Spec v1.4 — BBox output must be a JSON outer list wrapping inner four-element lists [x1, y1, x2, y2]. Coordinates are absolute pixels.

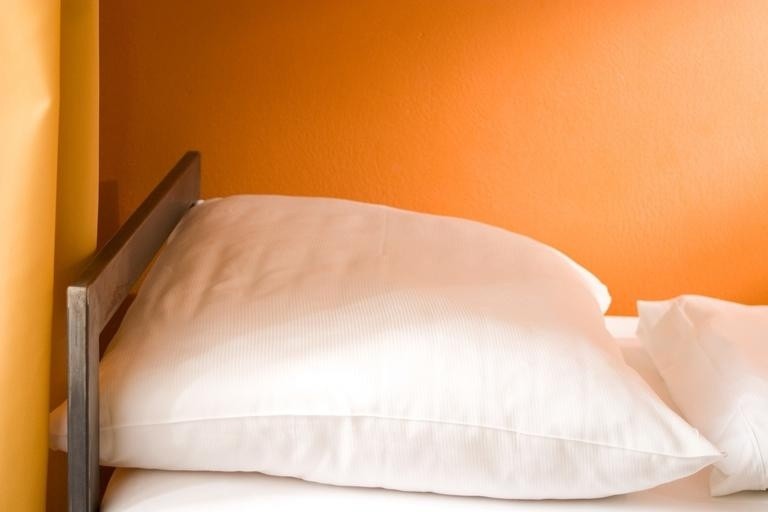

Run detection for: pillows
[[637, 295, 768, 496], [50, 194, 725, 497]]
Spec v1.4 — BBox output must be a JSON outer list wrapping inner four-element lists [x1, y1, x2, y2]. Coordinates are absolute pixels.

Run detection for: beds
[[67, 151, 767, 510]]
